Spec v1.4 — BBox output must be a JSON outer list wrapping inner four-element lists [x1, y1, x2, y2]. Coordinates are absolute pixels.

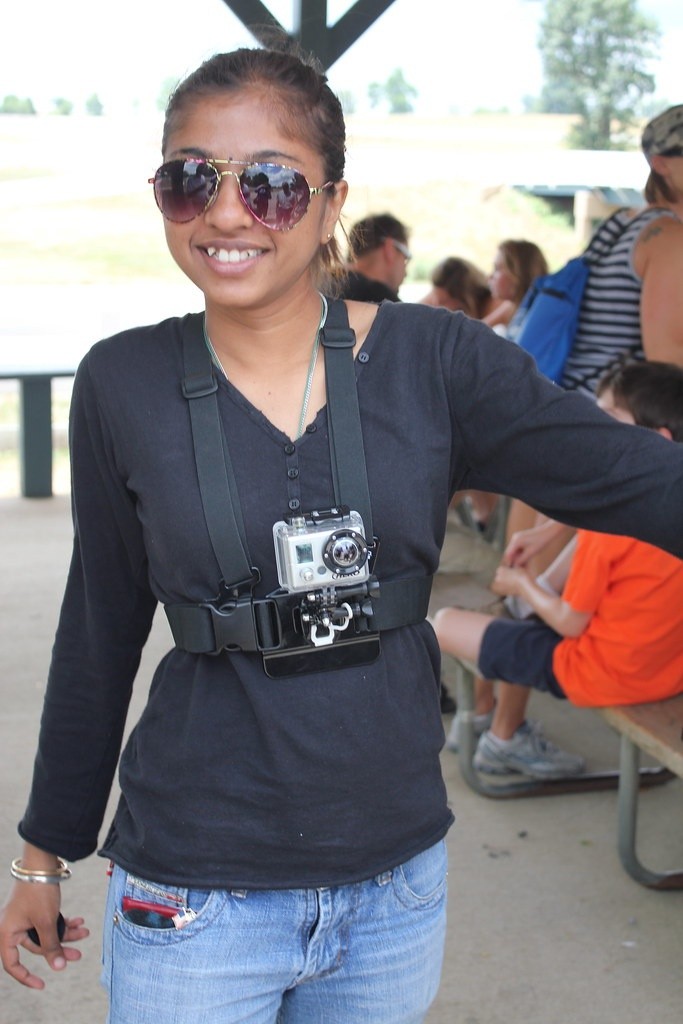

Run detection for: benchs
[[482, 602, 683, 891]]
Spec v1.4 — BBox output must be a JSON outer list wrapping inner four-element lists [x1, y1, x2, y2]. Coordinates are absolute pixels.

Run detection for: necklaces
[[202, 293, 328, 438]]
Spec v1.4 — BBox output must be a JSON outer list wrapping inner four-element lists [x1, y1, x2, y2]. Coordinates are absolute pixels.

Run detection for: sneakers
[[471, 724, 585, 778], [446, 711, 546, 752]]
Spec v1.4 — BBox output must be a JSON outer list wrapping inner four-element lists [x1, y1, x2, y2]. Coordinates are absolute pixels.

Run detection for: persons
[[420, 104, 683, 778], [329, 213, 408, 303], [0, 48, 683, 1024]]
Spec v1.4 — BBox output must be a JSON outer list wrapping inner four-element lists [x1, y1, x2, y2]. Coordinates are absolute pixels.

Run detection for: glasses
[[148, 159, 335, 232], [382, 237, 412, 266]]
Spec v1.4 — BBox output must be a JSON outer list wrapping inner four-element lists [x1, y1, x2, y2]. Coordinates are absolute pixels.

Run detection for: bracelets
[[10, 857, 71, 883]]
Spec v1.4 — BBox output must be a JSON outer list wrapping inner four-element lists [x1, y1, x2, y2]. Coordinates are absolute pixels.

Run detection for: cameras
[[272, 505, 370, 595]]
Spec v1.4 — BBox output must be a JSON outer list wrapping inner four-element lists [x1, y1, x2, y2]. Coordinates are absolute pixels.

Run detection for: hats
[[642, 105, 683, 159]]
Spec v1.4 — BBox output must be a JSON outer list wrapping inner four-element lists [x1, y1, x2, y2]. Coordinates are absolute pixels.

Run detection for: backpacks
[[504, 213, 644, 385]]
